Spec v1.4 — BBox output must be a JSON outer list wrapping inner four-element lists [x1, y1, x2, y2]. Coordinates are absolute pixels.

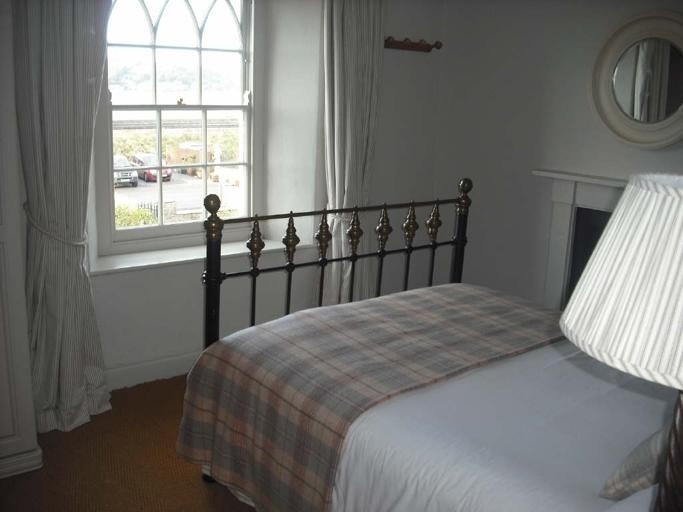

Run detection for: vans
[[130, 151, 172, 182]]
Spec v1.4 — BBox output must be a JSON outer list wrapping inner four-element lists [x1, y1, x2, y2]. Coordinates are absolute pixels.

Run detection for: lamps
[[559, 175, 683, 512]]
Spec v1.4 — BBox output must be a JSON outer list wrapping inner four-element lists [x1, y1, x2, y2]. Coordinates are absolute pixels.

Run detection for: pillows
[[597, 415, 672, 502]]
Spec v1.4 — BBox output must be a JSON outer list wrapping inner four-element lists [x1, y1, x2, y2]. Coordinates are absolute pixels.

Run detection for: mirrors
[[591, 12, 683, 149]]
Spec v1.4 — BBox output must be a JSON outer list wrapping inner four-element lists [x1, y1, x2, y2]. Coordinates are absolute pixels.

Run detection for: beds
[[199, 179, 682, 512]]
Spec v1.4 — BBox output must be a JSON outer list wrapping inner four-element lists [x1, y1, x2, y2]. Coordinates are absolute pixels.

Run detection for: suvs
[[114, 154, 137, 187]]
